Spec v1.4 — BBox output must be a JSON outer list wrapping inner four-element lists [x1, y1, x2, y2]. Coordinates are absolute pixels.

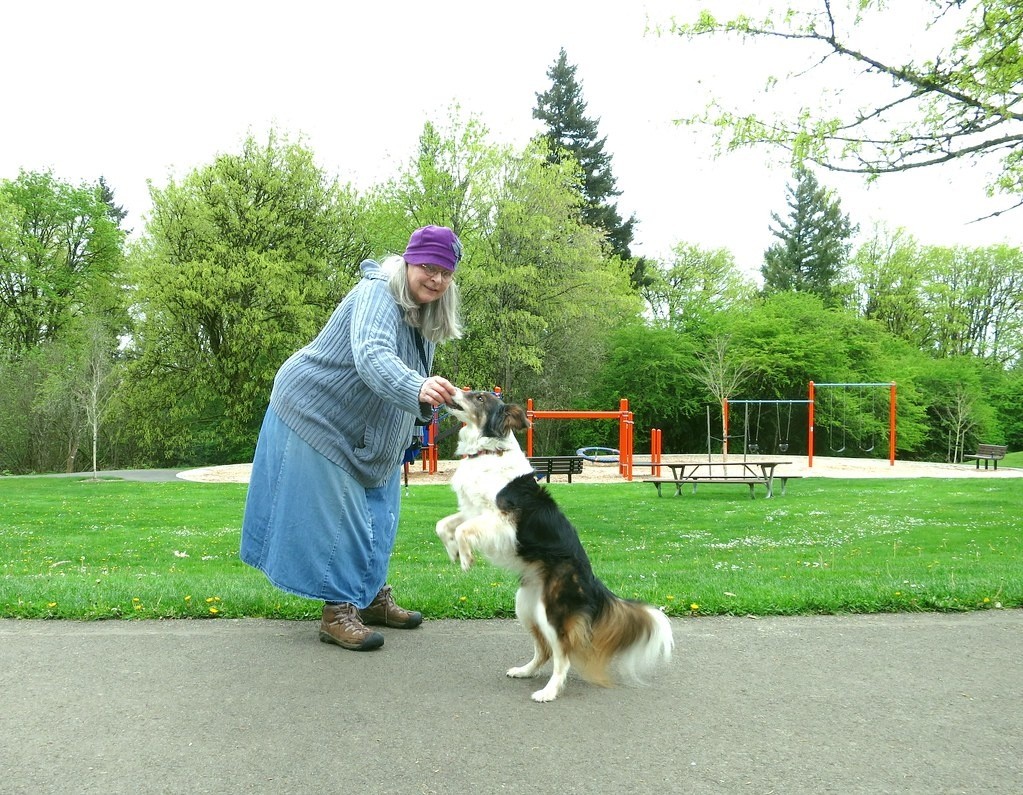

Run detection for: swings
[[830, 385, 846, 453], [747, 402, 762, 454], [859, 385, 875, 453], [776, 401, 792, 453]]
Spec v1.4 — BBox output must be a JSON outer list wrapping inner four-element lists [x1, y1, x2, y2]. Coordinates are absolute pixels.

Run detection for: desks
[[649, 461, 792, 499]]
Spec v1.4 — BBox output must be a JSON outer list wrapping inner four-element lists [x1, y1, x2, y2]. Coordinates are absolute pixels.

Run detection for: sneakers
[[319, 603, 384, 651], [357, 586, 422, 629]]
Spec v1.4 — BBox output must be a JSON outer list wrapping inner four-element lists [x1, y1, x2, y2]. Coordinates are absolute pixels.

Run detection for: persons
[[239, 224, 463, 649]]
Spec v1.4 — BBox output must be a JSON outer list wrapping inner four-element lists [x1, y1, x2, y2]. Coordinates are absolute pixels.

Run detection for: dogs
[[435, 386, 675, 702]]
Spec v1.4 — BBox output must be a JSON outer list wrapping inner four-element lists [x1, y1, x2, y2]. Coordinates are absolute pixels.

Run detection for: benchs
[[686, 475, 803, 497], [642, 478, 768, 500], [524, 456, 584, 484], [963, 443, 1007, 470]]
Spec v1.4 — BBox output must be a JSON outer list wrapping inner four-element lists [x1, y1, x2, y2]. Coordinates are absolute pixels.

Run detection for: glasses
[[422, 264, 453, 283]]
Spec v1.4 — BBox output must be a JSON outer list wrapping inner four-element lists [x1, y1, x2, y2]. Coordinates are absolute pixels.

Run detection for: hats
[[402, 225, 463, 272]]
